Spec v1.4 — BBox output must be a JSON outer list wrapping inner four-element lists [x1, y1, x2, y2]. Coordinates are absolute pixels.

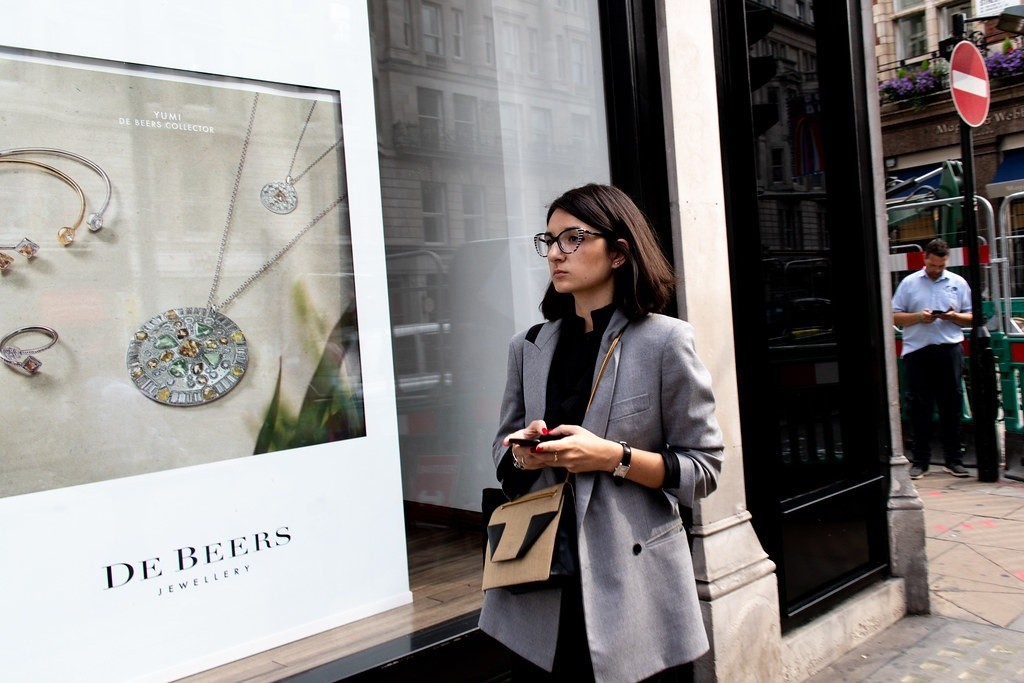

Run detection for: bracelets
[[917, 312, 922, 322], [514, 453, 523, 470], [0, 147, 112, 269]]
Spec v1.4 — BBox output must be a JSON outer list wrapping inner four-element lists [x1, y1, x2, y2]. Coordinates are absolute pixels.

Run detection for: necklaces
[[261, 100, 343, 214], [127, 93, 345, 406]]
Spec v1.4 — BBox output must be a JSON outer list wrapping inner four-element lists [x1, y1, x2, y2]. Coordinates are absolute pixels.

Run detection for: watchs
[[613, 441, 632, 481]]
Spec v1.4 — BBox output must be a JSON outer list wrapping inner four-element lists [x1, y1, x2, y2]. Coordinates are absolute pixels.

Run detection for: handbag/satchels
[[482, 483, 563, 589]]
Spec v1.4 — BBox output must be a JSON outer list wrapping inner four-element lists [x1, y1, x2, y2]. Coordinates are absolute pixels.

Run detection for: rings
[[554, 452, 559, 462], [522, 456, 526, 465], [0, 325, 59, 373]]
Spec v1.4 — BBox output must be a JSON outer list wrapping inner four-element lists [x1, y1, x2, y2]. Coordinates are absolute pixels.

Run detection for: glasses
[[535, 228, 608, 257]]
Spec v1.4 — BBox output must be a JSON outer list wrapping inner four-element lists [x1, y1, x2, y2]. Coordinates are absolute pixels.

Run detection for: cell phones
[[509, 438, 542, 448], [932, 310, 943, 316]]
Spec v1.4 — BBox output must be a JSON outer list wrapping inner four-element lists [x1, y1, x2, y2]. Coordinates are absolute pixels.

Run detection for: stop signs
[[948, 39, 991, 128]]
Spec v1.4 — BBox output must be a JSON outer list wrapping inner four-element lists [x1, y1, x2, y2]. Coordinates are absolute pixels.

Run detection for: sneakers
[[909, 466, 930, 478], [943, 461, 970, 475]]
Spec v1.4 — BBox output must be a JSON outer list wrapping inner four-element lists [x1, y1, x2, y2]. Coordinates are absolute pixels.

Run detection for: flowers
[[878, 60, 947, 111], [983, 37, 1024, 76]]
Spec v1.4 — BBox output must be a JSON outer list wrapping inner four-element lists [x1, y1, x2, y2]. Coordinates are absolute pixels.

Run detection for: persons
[[891, 240, 973, 477], [477, 184, 725, 683]]
